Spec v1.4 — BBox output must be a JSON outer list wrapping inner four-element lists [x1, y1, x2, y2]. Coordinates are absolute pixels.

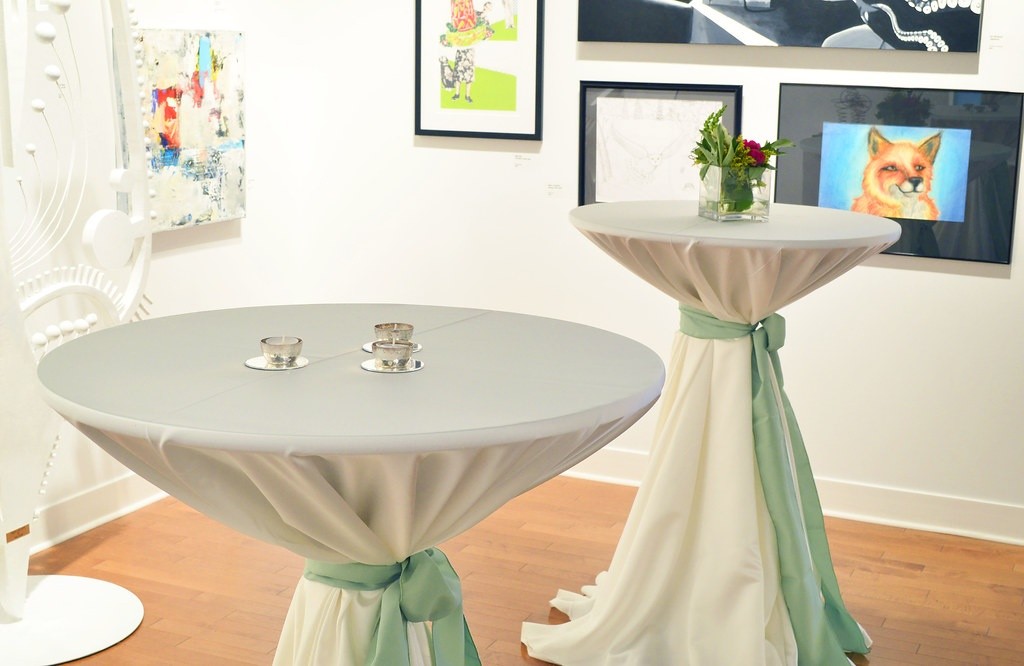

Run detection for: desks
[[35, 302, 661, 666], [523, 205, 907, 666]]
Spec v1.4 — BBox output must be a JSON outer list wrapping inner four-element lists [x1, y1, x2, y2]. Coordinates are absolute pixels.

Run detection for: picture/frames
[[414, 0, 544, 142], [576, 81, 744, 207]]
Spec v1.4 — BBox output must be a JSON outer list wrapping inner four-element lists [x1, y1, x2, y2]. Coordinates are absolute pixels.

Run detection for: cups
[[374, 322, 415, 341], [260, 336, 303, 370], [372, 340, 414, 372]]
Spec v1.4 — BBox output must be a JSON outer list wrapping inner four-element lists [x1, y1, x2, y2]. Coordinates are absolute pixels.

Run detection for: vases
[[697, 163, 772, 221]]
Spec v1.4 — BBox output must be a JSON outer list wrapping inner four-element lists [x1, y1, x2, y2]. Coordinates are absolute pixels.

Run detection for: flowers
[[691, 106, 796, 214]]
